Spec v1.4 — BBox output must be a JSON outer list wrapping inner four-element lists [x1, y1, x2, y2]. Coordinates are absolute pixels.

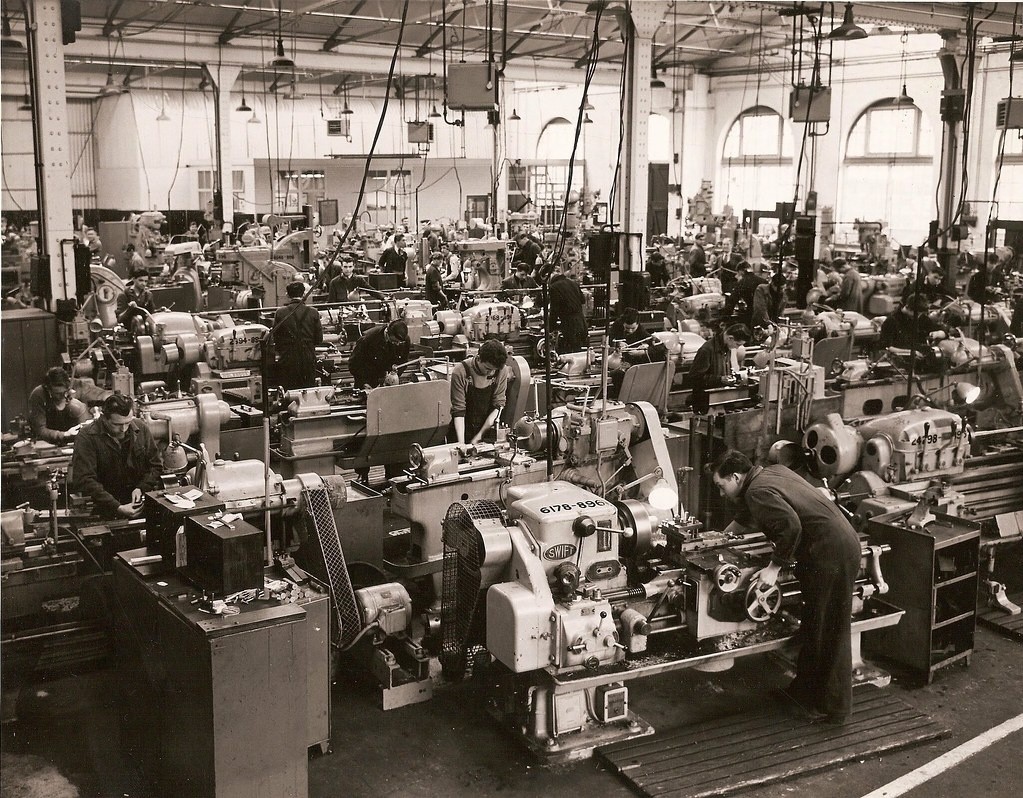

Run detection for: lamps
[[1009, 47, 1023, 61], [428, 80, 441, 117], [236, 72, 252, 111], [156, 79, 170, 121], [651, 69, 666, 88], [17, 84, 32, 111], [341, 85, 353, 113], [508, 88, 521, 120], [828, 1, 868, 40], [266, 0, 297, 69], [579, 94, 594, 110], [894, 61, 914, 104], [100, 36, 121, 94], [583, 113, 592, 122], [1, 0, 23, 48]]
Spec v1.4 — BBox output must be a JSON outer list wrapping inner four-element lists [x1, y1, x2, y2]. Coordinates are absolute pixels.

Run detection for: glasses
[[387, 332, 406, 344]]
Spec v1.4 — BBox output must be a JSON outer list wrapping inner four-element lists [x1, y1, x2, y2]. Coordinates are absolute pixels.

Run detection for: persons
[[117, 268, 156, 331], [273, 282, 323, 449], [539, 264, 590, 354], [348, 319, 410, 391], [645, 224, 1022, 375], [447, 339, 508, 446], [710, 449, 861, 724], [27, 366, 89, 447], [327, 230, 545, 321], [687, 323, 751, 530], [605, 307, 668, 398], [72, 394, 163, 616]]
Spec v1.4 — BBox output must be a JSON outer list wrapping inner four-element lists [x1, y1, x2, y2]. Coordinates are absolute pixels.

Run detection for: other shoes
[[773, 685, 810, 700], [793, 706, 845, 725]]
